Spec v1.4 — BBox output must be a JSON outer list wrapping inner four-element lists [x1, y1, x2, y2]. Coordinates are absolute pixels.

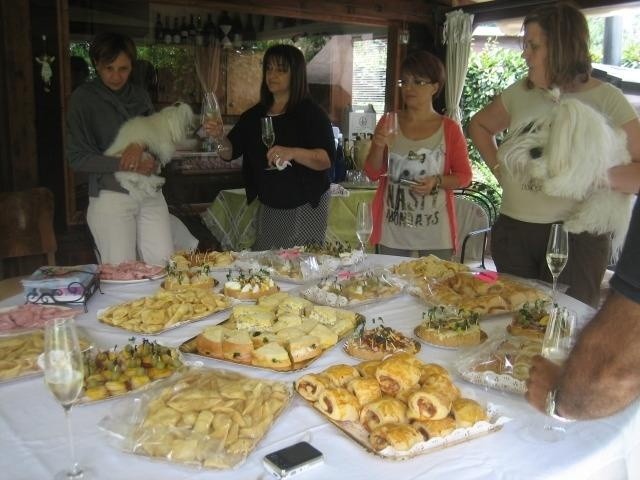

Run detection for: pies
[[295, 352, 487, 452]]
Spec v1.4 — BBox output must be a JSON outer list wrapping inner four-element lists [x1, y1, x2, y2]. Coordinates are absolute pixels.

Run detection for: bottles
[[334, 136, 362, 183]]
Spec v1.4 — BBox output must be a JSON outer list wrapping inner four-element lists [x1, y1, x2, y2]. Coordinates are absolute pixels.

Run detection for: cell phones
[[263, 440, 324, 474]]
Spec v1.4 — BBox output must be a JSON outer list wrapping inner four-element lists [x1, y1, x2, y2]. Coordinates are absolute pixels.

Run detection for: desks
[[218, 183, 383, 253]]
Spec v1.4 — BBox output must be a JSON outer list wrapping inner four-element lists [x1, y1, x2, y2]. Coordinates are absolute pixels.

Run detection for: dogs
[[103, 101, 193, 202], [496, 99, 635, 265]]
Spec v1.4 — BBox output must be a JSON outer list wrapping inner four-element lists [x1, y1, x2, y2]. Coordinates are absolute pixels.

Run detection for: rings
[[376, 124, 384, 129], [275, 154, 280, 158], [394, 131, 398, 136], [128, 164, 135, 168], [387, 127, 393, 133]]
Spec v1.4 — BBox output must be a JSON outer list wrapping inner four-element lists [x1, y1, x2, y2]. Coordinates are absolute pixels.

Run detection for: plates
[[456, 332, 566, 402], [1, 335, 94, 380], [161, 279, 218, 289], [342, 337, 423, 359], [417, 290, 548, 318], [178, 309, 365, 371], [96, 291, 241, 334], [98, 263, 165, 283], [2, 305, 70, 335], [250, 254, 339, 285], [302, 269, 407, 308], [505, 316, 566, 338], [385, 255, 462, 296], [220, 286, 281, 301], [295, 360, 515, 461], [414, 322, 489, 350]]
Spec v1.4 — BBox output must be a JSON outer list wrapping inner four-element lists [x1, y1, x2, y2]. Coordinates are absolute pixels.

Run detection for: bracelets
[[492, 163, 501, 175], [428, 173, 442, 196]]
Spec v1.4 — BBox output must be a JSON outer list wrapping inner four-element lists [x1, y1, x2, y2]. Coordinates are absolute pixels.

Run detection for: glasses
[[395, 77, 434, 89]]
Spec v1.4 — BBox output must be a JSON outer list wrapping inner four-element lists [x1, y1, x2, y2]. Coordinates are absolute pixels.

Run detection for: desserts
[[164, 268, 544, 358]]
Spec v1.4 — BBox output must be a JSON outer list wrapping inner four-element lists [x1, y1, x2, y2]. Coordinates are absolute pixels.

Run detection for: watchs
[[543, 387, 578, 423]]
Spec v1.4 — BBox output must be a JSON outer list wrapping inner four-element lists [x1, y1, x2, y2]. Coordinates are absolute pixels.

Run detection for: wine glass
[[345, 169, 369, 186], [204, 90, 228, 154], [526, 308, 579, 444], [381, 112, 399, 178], [261, 116, 280, 171], [40, 316, 98, 480], [545, 222, 569, 304], [355, 198, 374, 258]]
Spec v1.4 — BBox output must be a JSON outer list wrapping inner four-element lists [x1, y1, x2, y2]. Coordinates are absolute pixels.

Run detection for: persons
[[130, 58, 160, 113], [63, 29, 175, 264], [467, 3, 640, 309], [362, 49, 473, 261], [524, 194, 640, 422], [69, 56, 90, 94], [203, 43, 336, 252]]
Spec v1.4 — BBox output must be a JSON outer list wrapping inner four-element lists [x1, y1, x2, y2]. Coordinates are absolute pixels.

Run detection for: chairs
[[1, 184, 60, 280], [451, 181, 502, 270]]
